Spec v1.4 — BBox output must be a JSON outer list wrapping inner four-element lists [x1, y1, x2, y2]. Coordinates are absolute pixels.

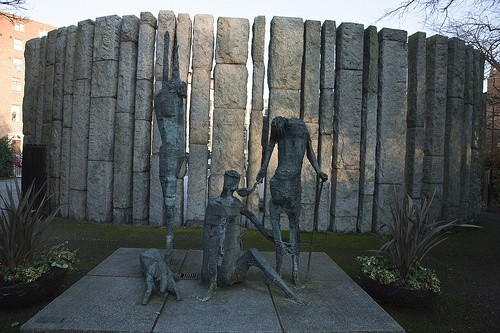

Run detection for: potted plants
[[353, 178, 485, 309], [0, 176, 60, 307], [32, 243, 79, 286]]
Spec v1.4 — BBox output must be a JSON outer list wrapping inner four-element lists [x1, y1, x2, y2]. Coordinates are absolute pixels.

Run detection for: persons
[[256, 117, 326, 288], [197, 170, 307, 305]]
